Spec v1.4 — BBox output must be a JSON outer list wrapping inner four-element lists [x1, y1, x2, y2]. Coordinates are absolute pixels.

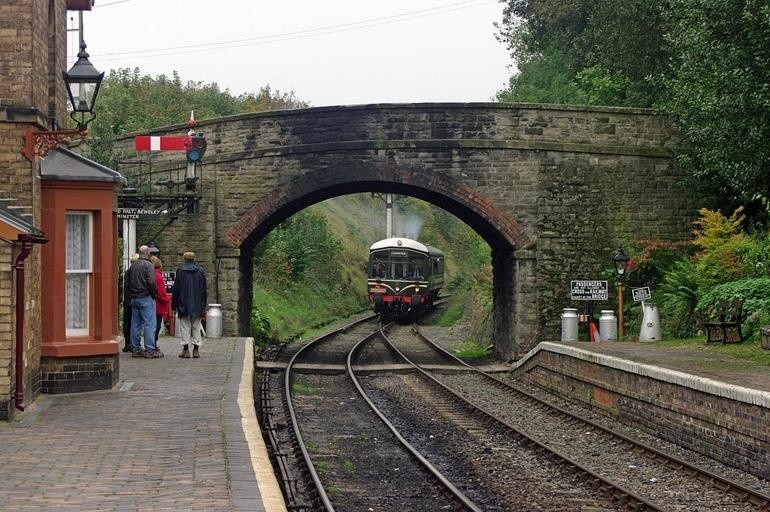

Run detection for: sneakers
[[192, 349, 200, 357], [123, 344, 164, 358], [178, 350, 189, 358]]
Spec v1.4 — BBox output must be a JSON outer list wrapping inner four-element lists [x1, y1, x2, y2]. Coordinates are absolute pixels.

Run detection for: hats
[[184, 252, 194, 259]]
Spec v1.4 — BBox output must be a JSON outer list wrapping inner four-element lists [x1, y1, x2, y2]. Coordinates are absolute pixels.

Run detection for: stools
[[760, 324, 770, 350]]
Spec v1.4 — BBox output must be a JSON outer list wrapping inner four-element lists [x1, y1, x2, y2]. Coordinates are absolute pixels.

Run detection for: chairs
[[703, 300, 745, 346]]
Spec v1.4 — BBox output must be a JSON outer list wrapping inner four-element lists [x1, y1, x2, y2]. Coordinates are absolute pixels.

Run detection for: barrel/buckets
[[175, 312, 183, 337], [205, 304, 223, 337], [561, 308, 579, 343], [599, 310, 618, 341]]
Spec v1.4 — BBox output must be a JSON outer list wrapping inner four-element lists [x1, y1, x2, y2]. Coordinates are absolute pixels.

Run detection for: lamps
[[62, 43, 105, 132]]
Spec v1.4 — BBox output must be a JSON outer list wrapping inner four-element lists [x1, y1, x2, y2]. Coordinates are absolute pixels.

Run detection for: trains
[[368, 238, 444, 319]]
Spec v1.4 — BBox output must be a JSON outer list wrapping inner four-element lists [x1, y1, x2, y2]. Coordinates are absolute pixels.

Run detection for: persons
[[121, 253, 141, 353], [171, 252, 208, 359], [411, 262, 424, 279], [434, 259, 440, 267], [148, 254, 170, 350], [128, 244, 165, 359]]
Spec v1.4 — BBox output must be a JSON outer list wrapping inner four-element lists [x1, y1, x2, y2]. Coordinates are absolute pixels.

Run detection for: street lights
[[613, 246, 630, 343]]
